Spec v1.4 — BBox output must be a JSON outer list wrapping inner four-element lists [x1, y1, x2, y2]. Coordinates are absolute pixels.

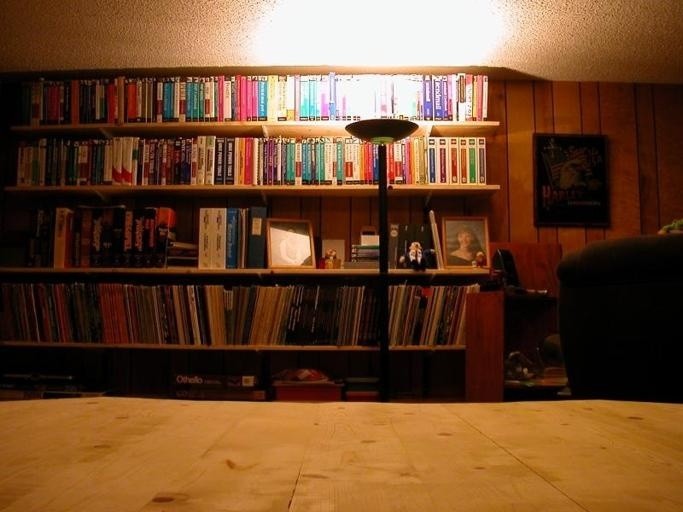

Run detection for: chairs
[[556, 233, 682, 403]]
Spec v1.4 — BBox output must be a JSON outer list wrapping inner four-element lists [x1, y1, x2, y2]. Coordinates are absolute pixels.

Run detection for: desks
[[0, 398, 683, 512]]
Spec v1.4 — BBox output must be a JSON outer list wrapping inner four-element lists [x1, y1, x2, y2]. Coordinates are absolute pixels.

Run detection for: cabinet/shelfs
[[0, 64, 506, 401]]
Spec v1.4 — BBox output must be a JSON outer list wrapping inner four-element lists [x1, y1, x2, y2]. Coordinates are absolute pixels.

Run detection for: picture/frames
[[264, 218, 317, 269], [529, 132, 615, 230], [441, 217, 489, 267]]
